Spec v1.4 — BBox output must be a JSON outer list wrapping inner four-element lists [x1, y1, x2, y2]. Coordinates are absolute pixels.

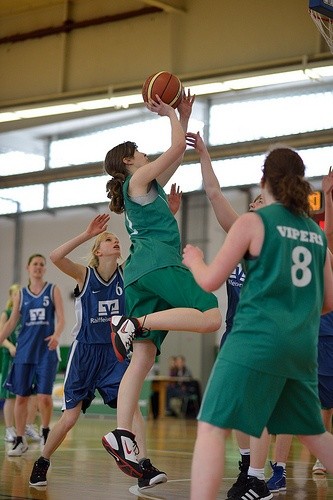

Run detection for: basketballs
[[141, 70, 183, 115]]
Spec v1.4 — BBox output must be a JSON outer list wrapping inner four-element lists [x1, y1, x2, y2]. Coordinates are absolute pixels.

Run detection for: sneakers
[[110, 314, 151, 362], [226, 454, 250, 497], [227, 476, 274, 500], [5, 429, 15, 441], [29, 457, 51, 485], [8, 436, 29, 457], [266, 461, 286, 492], [137, 459, 169, 490], [312, 460, 327, 474], [101, 428, 142, 477], [25, 424, 41, 440], [39, 426, 51, 451]]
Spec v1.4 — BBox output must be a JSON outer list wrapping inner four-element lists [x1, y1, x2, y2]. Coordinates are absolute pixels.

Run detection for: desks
[[149, 376, 194, 419]]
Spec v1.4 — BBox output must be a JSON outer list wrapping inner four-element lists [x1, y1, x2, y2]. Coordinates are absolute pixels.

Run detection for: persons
[[103, 87, 221, 479], [0, 255, 63, 457], [150, 357, 192, 417], [181, 133, 333, 500], [29, 183, 182, 491]]
